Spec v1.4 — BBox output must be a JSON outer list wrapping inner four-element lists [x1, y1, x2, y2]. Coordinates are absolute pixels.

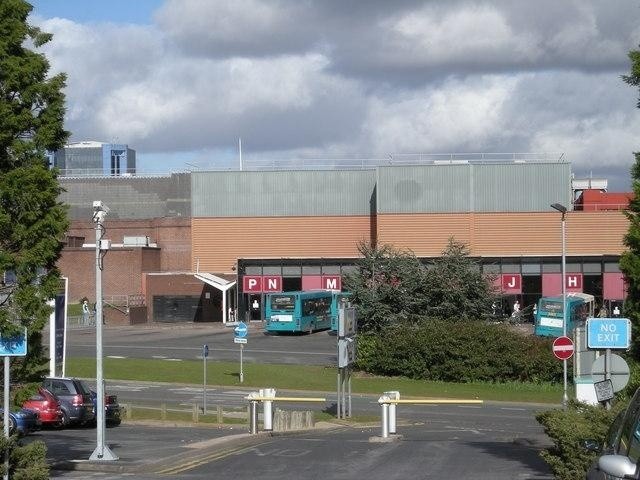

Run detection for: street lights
[[551, 203, 570, 411]]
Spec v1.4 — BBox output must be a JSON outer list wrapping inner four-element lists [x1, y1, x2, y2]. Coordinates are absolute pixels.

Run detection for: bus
[[329, 290, 354, 335], [264, 287, 341, 335], [534, 291, 596, 340]]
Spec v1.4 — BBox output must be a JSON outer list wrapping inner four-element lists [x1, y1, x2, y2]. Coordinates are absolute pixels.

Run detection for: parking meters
[[258, 388, 278, 431], [383, 390, 401, 434]]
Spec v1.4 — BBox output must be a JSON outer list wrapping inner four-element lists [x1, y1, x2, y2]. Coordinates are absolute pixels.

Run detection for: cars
[[88, 388, 122, 428], [580, 408, 627, 480], [508, 302, 535, 326], [1, 403, 42, 439]]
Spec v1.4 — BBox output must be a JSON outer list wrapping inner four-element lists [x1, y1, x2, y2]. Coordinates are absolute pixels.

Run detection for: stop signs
[[551, 335, 575, 360]]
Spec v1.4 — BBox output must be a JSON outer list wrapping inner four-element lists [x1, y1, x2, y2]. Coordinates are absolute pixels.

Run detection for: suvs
[[597, 385, 640, 480], [0, 382, 65, 432], [34, 377, 96, 429]]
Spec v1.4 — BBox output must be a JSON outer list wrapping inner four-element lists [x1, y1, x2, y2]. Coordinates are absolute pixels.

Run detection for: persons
[[83, 300, 105, 324], [513, 300, 522, 327], [532, 303, 538, 326], [571, 304, 620, 320]]
